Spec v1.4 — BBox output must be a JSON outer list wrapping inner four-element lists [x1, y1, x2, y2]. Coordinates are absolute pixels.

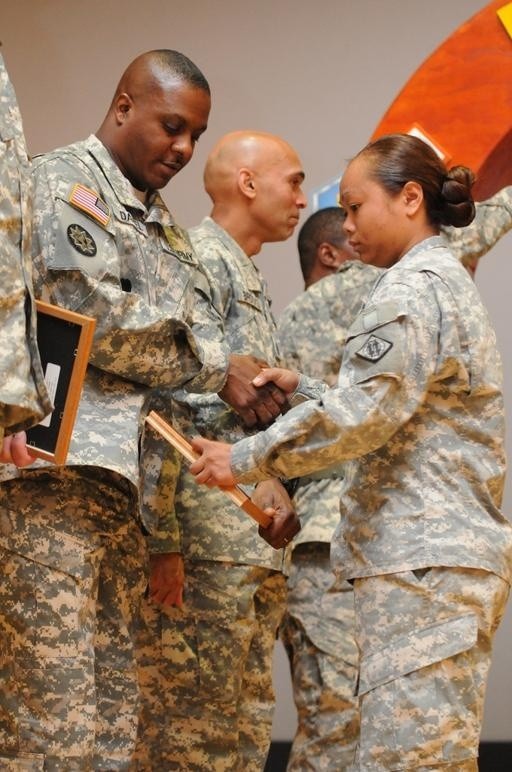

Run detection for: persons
[[1, 41, 304, 771], [182, 127, 510, 771], [0, 53, 56, 496], [271, 166, 510, 770], [146, 124, 310, 771]]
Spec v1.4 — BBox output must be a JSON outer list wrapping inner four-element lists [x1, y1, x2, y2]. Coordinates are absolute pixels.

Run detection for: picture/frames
[[13, 299, 97, 466], [144, 406, 277, 532]]
